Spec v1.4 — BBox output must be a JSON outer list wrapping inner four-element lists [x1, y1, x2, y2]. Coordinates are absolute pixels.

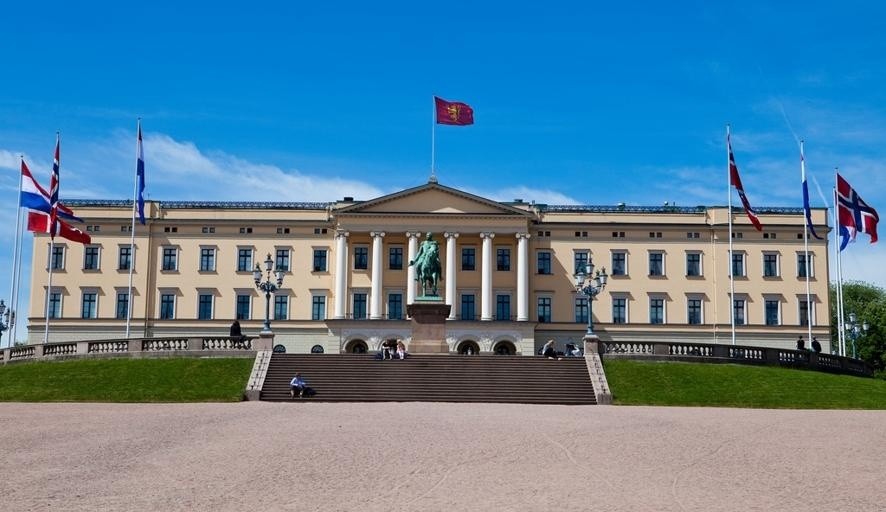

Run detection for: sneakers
[[548, 357, 563, 361], [383, 357, 404, 360]]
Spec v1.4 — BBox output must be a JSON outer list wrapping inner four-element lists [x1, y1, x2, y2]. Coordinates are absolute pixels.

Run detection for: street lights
[[0, 298, 15, 346], [251, 252, 285, 332], [844, 313, 869, 359], [575, 258, 608, 335]]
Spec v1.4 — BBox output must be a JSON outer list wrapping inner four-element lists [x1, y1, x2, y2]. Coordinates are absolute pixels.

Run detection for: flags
[[18, 158, 83, 222], [835, 171, 880, 244], [833, 188, 856, 251], [799, 145, 824, 241], [433, 93, 475, 126], [46, 138, 59, 242], [26, 207, 90, 246], [726, 134, 761, 231], [136, 124, 145, 225]]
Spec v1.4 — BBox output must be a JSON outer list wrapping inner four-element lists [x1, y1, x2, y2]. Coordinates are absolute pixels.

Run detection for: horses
[[420, 241, 440, 298]]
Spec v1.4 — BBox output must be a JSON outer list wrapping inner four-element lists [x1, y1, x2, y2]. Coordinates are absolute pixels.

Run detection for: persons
[[406, 231, 443, 282], [542, 340, 563, 361], [380, 338, 393, 361], [809, 335, 821, 353], [793, 334, 804, 352], [287, 372, 306, 400], [562, 337, 576, 357], [395, 338, 406, 361], [229, 320, 244, 343]]
